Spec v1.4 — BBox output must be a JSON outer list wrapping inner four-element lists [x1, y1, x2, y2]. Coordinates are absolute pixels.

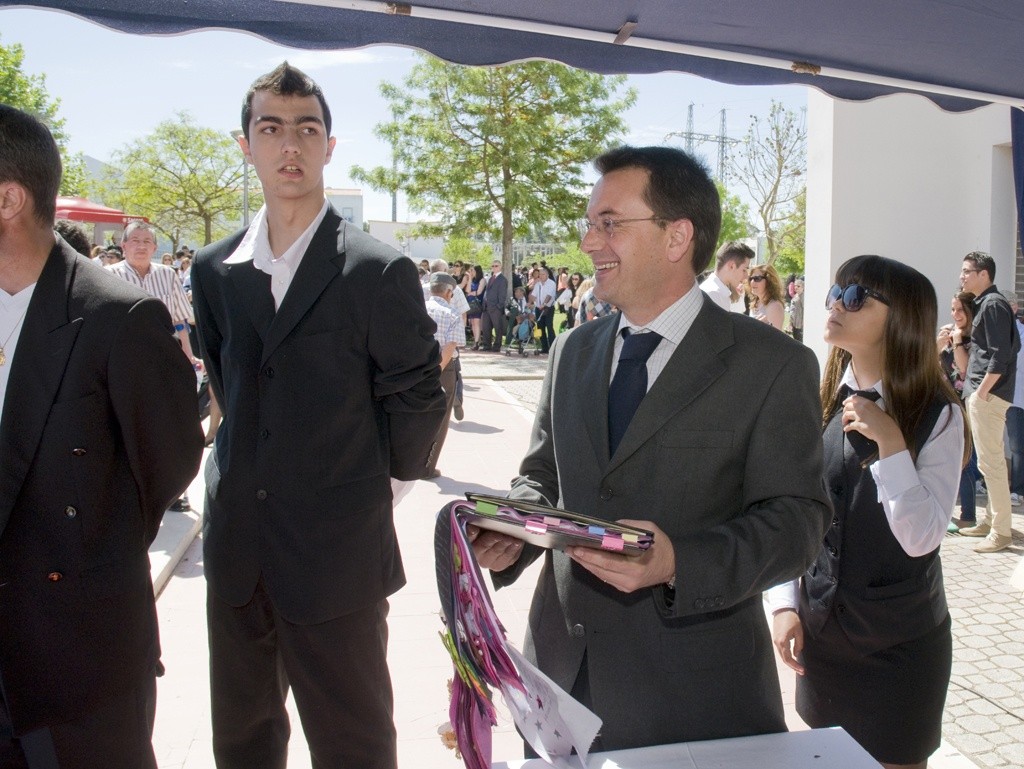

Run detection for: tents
[[54, 196, 149, 225]]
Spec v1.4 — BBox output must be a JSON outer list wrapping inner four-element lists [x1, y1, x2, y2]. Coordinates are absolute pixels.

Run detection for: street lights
[[229, 129, 249, 228]]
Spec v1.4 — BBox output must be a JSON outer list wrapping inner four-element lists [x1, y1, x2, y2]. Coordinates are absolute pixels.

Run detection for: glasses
[[826, 283, 890, 311], [571, 278, 578, 280], [453, 265, 459, 267], [748, 276, 766, 281], [492, 265, 499, 267], [961, 269, 977, 274], [448, 288, 454, 297], [577, 217, 665, 238]]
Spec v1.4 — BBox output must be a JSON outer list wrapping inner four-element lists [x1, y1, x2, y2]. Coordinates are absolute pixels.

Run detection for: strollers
[[505, 305, 547, 358]]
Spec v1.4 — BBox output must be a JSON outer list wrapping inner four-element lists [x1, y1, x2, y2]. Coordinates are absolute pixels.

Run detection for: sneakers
[[975, 479, 988, 496], [959, 522, 992, 536], [1010, 493, 1023, 506], [973, 531, 1012, 552]]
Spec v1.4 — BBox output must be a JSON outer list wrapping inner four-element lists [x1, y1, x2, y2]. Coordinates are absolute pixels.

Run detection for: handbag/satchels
[[466, 290, 482, 314]]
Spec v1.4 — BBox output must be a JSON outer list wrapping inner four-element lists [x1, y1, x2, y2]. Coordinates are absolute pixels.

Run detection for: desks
[[489, 725, 886, 769]]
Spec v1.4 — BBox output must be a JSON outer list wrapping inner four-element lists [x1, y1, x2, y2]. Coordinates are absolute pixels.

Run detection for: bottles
[[194, 364, 202, 393]]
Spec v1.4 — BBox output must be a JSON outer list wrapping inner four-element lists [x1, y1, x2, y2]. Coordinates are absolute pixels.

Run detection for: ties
[[843, 389, 881, 461], [607, 325, 661, 459], [488, 276, 495, 288]]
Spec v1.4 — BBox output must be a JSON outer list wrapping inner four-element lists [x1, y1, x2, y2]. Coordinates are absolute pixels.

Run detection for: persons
[[695, 241, 805, 344], [765, 254, 964, 769], [53, 221, 222, 514], [191, 60, 448, 769], [464, 145, 833, 759], [959, 251, 1022, 552], [997, 290, 1024, 506], [937, 288, 980, 532], [412, 259, 619, 422], [0, 102, 204, 769]]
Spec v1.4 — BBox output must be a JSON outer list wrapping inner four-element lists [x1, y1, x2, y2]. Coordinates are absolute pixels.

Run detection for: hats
[[431, 271, 456, 290], [514, 287, 526, 295]]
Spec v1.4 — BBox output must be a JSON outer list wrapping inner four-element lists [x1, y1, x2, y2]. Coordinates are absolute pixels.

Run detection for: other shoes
[[171, 499, 191, 512], [540, 349, 549, 355], [946, 519, 976, 532], [480, 349, 491, 351], [453, 398, 464, 420], [490, 349, 499, 352], [471, 343, 479, 350]]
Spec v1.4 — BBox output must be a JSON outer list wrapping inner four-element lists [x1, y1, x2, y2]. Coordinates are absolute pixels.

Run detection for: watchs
[[543, 303, 546, 305], [951, 342, 963, 350]]
